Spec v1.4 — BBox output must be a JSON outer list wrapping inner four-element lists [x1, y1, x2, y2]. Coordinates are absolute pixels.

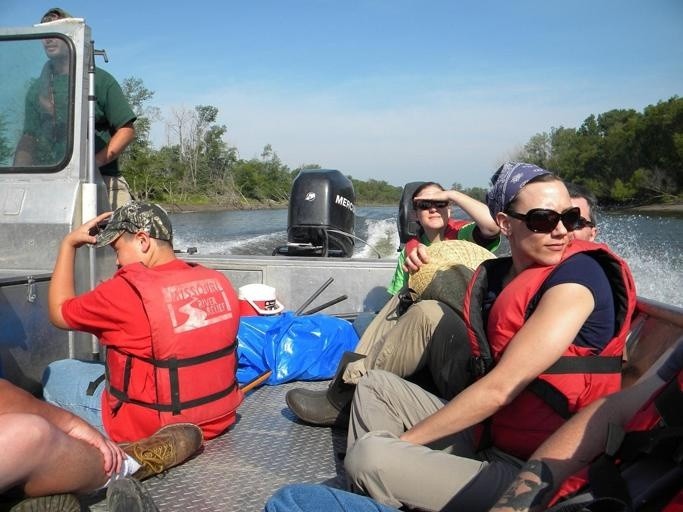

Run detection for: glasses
[[507, 207, 580, 234], [415, 199, 449, 209]]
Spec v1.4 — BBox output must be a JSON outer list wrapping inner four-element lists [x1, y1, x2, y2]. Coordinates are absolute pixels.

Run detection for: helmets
[[577, 216, 594, 229]]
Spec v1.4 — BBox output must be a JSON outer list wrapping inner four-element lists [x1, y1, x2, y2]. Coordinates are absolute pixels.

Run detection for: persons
[[43, 200, 243, 454], [12, 6, 142, 215], [335, 154, 638, 510], [0, 369, 205, 498], [350, 179, 504, 346], [283, 190, 600, 429], [262, 332, 682, 512]]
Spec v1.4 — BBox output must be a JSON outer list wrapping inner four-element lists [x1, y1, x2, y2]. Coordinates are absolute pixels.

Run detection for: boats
[[1, 18, 681, 511]]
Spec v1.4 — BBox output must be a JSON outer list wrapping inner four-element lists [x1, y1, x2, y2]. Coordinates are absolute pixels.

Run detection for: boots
[[286, 351, 366, 425]]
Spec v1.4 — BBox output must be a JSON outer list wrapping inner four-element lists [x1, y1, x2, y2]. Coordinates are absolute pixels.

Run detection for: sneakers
[[7, 494, 80, 512], [106, 476, 157, 512], [118, 423, 204, 480]]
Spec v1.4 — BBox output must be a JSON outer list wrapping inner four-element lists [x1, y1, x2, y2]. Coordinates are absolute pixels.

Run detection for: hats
[[408, 240, 497, 318], [87, 201, 171, 249]]
